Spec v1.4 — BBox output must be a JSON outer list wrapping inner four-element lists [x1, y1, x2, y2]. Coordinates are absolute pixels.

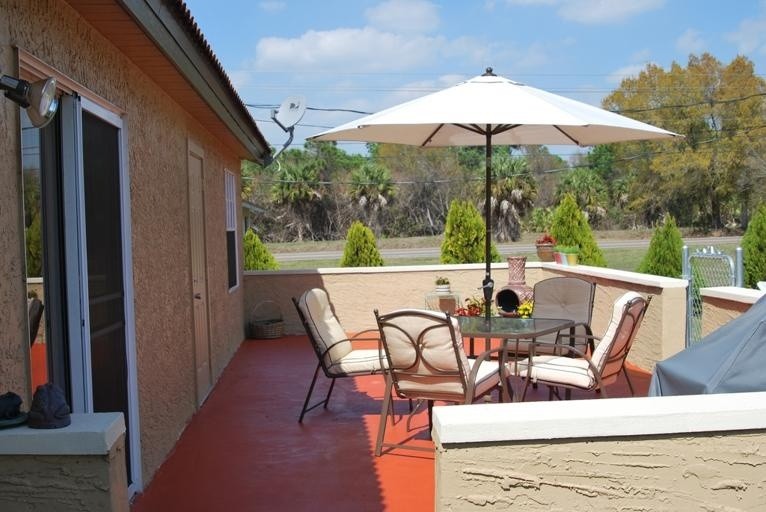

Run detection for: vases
[[536, 244, 556, 262], [499, 254, 534, 313]]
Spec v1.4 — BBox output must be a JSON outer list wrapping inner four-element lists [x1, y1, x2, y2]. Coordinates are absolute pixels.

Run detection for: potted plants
[[553, 246, 580, 266]]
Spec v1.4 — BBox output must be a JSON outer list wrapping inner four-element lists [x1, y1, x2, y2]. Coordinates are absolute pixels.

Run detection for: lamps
[[0, 75, 60, 128]]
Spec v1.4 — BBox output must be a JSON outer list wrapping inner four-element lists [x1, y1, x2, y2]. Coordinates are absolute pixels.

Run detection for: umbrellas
[[306, 67, 686, 360]]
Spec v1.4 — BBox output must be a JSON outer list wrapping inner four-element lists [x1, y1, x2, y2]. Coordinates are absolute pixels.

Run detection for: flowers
[[536, 234, 558, 245]]
